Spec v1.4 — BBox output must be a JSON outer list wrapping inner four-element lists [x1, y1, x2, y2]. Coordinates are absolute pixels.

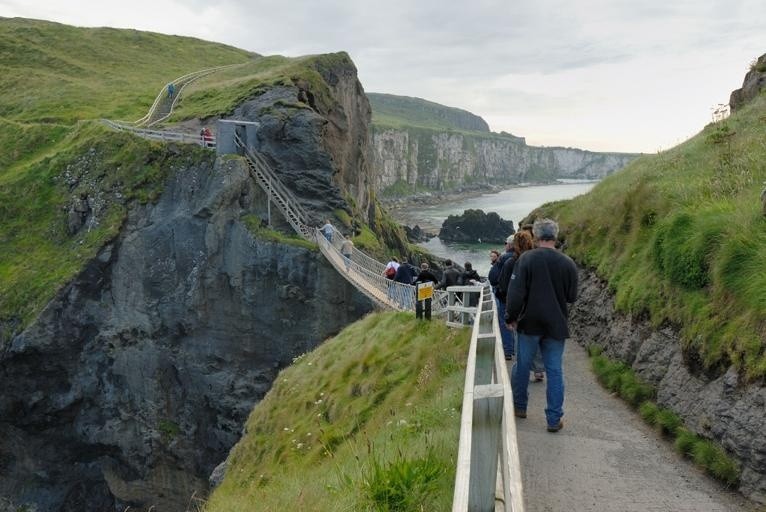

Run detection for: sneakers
[[514, 411, 527, 419], [534, 371, 544, 380], [548, 419, 563, 433]]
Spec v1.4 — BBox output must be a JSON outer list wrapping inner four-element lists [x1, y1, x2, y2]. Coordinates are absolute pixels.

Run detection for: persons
[[393, 256, 414, 311], [199, 128, 205, 140], [339, 234, 354, 273], [204, 128, 213, 147], [168, 82, 174, 99], [382, 256, 401, 302], [457, 260, 481, 324], [506, 217, 581, 433], [318, 219, 339, 251], [488, 223, 546, 381], [433, 258, 462, 307], [412, 262, 439, 287]]
